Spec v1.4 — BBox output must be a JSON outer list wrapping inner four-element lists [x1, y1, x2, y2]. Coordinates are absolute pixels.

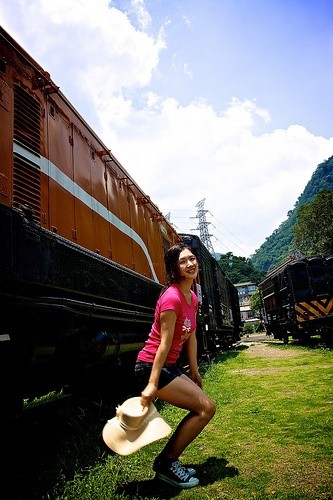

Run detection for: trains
[[0, 26, 240, 411], [255, 255, 333, 346]]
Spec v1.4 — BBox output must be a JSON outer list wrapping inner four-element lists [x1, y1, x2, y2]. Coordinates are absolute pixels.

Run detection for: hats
[[102, 397, 172, 456]]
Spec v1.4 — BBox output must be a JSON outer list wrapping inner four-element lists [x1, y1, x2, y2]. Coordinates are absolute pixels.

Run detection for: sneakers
[[153, 454, 196, 475], [157, 458, 200, 487]]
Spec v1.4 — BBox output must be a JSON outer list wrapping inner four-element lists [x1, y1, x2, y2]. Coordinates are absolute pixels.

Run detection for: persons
[[136, 242, 216, 488]]
